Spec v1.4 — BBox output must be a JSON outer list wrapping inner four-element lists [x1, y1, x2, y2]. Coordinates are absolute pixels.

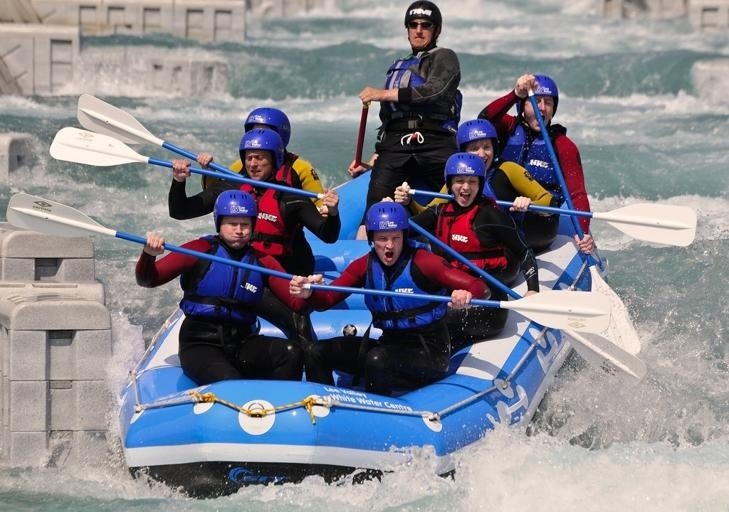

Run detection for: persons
[[169, 133, 342, 279], [347, 1, 463, 243], [135, 189, 324, 384], [288, 200, 492, 397], [395, 120, 553, 253], [394, 151, 540, 356], [476, 74, 596, 258], [195, 106, 329, 217]]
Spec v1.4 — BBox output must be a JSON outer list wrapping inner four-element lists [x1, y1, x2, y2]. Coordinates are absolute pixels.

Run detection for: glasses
[[407, 22, 431, 28]]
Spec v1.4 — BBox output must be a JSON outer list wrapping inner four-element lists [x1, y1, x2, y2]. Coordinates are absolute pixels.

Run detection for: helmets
[[516, 73, 559, 117], [245, 107, 290, 147], [404, 0, 442, 30], [365, 202, 411, 245], [456, 119, 501, 155], [214, 190, 258, 232], [239, 128, 285, 170], [445, 153, 485, 191]]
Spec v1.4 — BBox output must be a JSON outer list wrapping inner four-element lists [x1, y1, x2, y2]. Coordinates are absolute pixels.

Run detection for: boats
[[116, 168, 602, 501]]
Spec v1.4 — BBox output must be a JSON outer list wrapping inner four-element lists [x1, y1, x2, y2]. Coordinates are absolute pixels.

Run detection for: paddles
[[77, 93, 245, 176], [408, 218, 646, 389], [50, 127, 324, 200], [527, 88, 641, 354], [409, 188, 696, 245], [300, 283, 610, 332], [6, 193, 325, 284]]
